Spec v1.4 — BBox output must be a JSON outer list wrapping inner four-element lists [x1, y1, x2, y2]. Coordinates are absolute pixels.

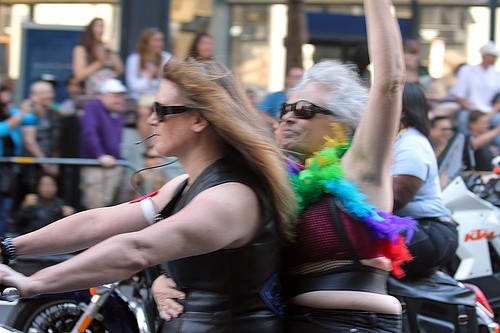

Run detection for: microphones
[[136, 133, 156, 144]]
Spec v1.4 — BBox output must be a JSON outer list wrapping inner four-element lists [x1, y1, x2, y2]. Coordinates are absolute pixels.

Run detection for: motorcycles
[[0, 254, 167, 333]]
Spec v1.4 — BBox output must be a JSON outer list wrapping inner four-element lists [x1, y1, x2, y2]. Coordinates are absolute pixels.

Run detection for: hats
[[97, 78, 127, 94], [480, 42, 499, 57]]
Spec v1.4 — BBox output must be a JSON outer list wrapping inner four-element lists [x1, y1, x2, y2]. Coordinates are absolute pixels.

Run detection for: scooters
[[384, 156, 499, 332]]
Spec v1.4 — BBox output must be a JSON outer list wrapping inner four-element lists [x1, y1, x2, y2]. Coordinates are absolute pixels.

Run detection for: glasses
[[1, 99, 13, 108], [152, 102, 204, 122], [278, 100, 337, 121]]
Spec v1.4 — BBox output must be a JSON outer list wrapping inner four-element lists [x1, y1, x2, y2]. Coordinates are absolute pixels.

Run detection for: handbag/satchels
[[387, 273, 479, 333]]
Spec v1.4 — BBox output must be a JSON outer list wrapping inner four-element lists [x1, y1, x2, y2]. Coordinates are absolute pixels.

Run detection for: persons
[[0, 72, 82, 239], [75, 79, 127, 209], [0, 58, 298, 332], [71, 18, 125, 84], [402, 40, 422, 83], [256, 62, 304, 121], [427, 42, 500, 208], [392, 83, 459, 280], [131, 144, 168, 201], [150, 0, 406, 332], [188, 32, 216, 60], [124, 25, 174, 104]]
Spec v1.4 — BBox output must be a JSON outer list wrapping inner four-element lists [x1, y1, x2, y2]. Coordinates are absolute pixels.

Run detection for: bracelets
[[3, 237, 16, 260]]
